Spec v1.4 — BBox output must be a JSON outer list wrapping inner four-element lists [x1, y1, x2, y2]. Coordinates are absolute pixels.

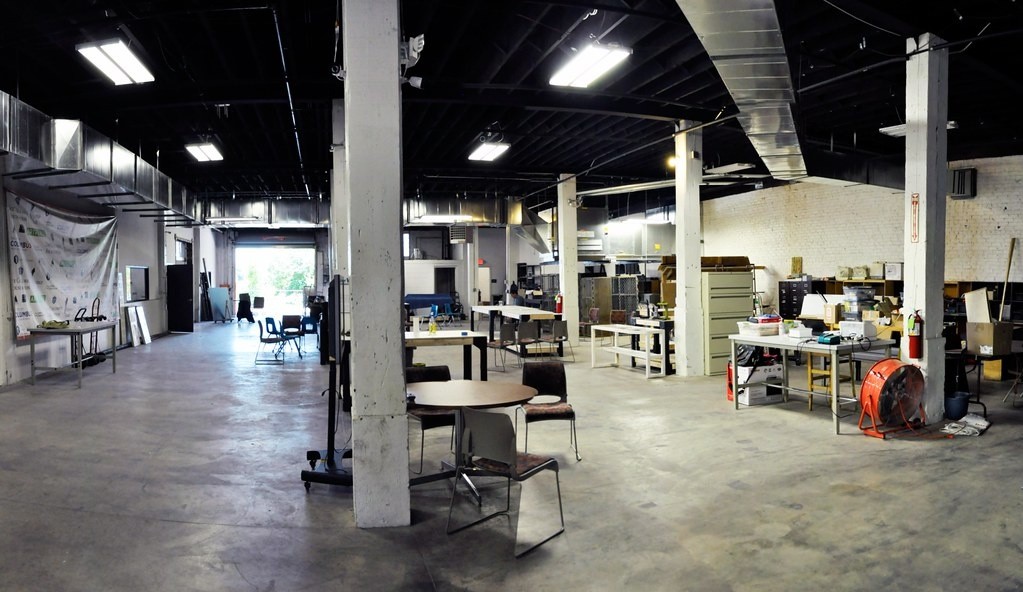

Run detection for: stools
[[808, 352, 858, 412]]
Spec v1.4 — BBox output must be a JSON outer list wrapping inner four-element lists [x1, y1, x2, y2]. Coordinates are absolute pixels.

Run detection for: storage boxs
[[885, 262, 904, 281], [966, 322, 1014, 356], [727, 363, 783, 405], [870, 263, 885, 280], [845, 299, 879, 312], [844, 286, 876, 301]]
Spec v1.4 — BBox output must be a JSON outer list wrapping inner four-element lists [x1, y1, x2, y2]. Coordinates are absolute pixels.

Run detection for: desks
[[406, 380, 538, 505], [471, 305, 564, 357], [405, 330, 488, 381], [26, 319, 118, 387], [728, 332, 896, 436]]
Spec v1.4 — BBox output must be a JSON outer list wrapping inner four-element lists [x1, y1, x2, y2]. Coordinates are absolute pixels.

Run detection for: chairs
[[514, 362, 582, 464], [255, 314, 319, 365], [580, 308, 604, 341], [441, 303, 462, 328], [419, 304, 442, 330], [404, 365, 456, 475], [610, 310, 631, 346], [503, 322, 544, 367], [538, 321, 576, 363], [445, 410, 566, 558], [488, 323, 521, 372]]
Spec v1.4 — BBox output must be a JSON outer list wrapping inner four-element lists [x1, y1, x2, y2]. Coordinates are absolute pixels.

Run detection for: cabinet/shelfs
[[779, 280, 1023, 354]]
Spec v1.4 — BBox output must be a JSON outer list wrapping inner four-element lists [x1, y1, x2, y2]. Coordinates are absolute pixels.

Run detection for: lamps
[[185, 133, 224, 161], [468, 131, 513, 161], [548, 39, 634, 88], [74, 29, 155, 86]]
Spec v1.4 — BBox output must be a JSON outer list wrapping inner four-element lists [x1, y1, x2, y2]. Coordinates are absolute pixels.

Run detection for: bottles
[[428, 312, 437, 335]]
